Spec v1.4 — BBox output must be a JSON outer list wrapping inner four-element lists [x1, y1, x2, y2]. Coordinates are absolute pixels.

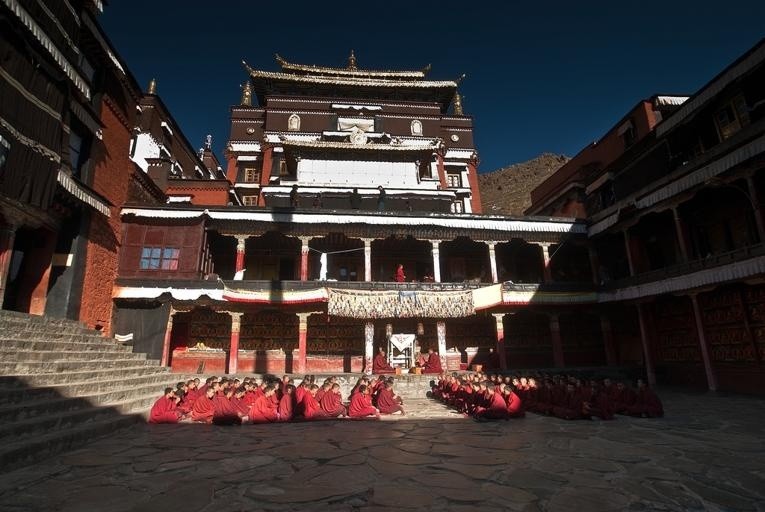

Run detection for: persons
[[290, 184, 301, 207], [424, 271, 434, 282], [422, 348, 441, 369], [351, 187, 361, 209], [395, 263, 407, 282], [149, 372, 404, 425], [431, 370, 665, 424], [377, 185, 385, 211], [373, 351, 393, 370], [312, 193, 321, 208]]
